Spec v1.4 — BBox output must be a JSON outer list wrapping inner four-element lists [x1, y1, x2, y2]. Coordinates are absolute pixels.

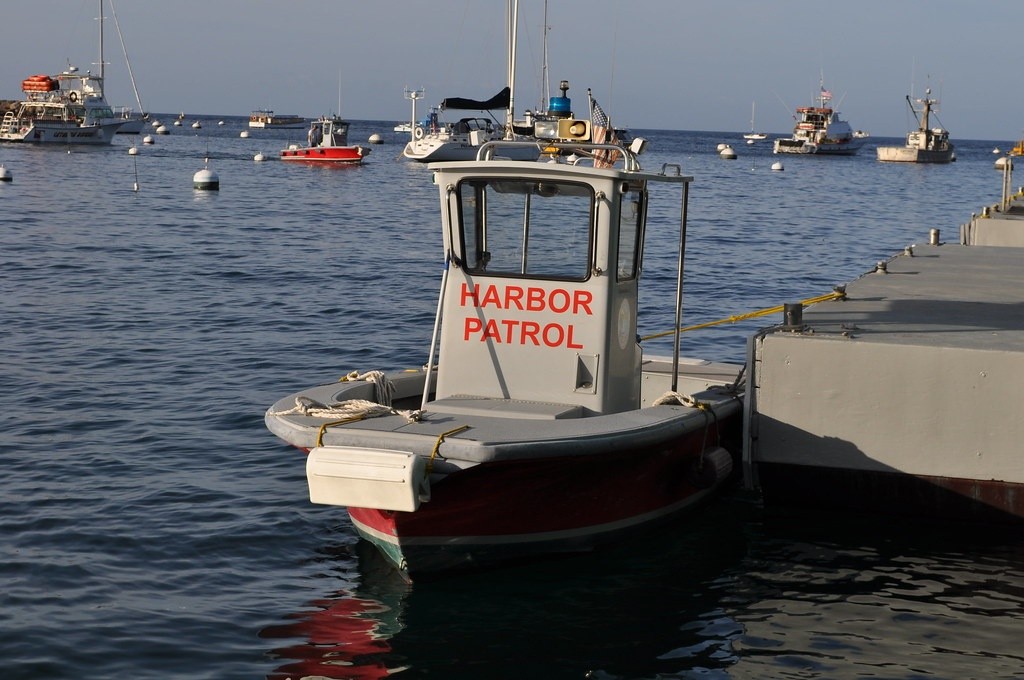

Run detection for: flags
[[820, 87, 832, 98], [589, 97, 623, 169]]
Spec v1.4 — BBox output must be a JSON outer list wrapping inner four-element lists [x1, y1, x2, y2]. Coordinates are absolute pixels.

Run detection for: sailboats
[[99, 1, 152, 134], [743, 100, 768, 140]]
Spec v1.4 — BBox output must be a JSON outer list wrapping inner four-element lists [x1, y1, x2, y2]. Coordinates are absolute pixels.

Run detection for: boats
[[403, 1, 632, 163], [0, 62, 136, 144], [875, 72, 955, 164], [394, 120, 419, 132], [248, 109, 306, 130], [262, 141, 716, 588], [773, 64, 870, 157], [277, 110, 374, 162]]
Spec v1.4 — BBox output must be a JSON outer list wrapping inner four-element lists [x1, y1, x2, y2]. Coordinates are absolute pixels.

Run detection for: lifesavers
[[415, 127, 423, 140], [28, 74, 50, 82], [252, 115, 257, 120], [69, 91, 78, 104]]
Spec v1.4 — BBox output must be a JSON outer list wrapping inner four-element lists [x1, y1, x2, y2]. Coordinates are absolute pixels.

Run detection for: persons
[[337, 125, 344, 132], [308, 124, 318, 147], [316, 125, 322, 145]]
